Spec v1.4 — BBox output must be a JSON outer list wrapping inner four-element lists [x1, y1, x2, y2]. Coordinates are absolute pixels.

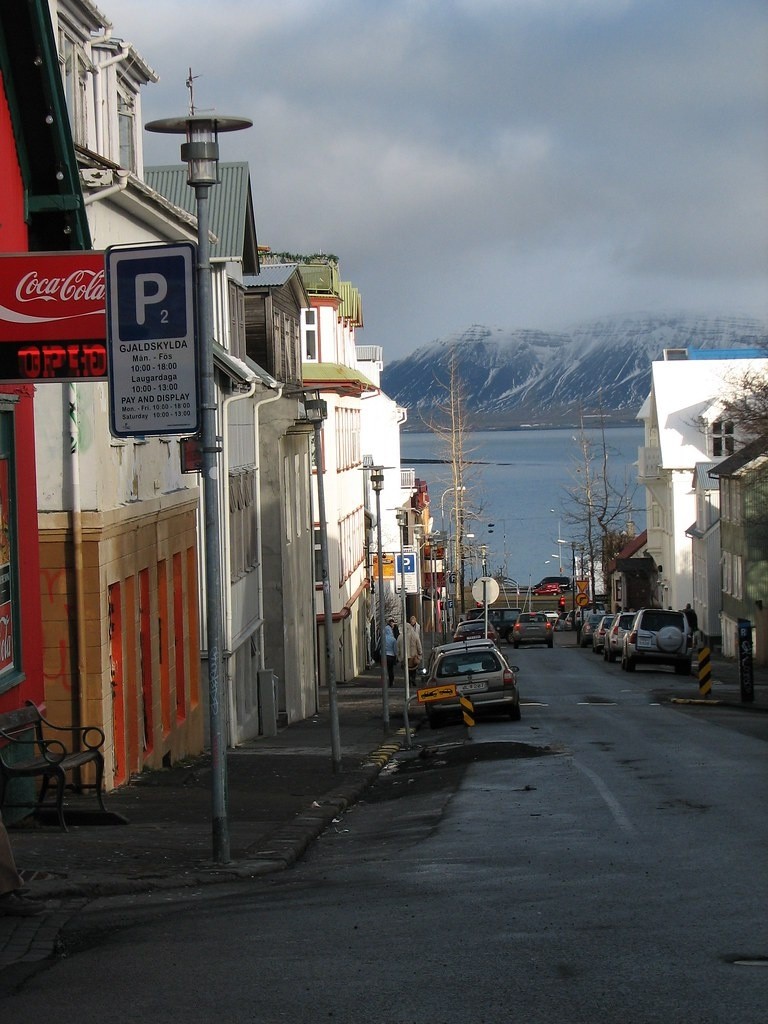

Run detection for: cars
[[428, 639, 509, 674], [552, 610, 575, 631], [423, 646, 521, 730], [580, 616, 602, 648], [531, 583, 561, 596], [604, 611, 637, 663], [593, 615, 616, 655], [453, 619, 501, 648], [512, 612, 554, 649]]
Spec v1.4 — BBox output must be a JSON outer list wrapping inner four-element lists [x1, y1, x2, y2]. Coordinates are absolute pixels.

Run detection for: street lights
[[557, 540, 592, 601], [357, 465, 396, 722], [432, 540, 443, 645], [422, 533, 440, 646], [441, 486, 466, 532], [568, 541, 577, 630], [144, 114, 255, 862]]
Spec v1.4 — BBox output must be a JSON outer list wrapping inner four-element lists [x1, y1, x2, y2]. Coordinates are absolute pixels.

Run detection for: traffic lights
[[560, 597, 565, 607]]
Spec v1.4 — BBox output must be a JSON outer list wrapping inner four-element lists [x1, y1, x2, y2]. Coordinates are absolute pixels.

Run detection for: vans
[[475, 608, 523, 645], [534, 577, 571, 590]]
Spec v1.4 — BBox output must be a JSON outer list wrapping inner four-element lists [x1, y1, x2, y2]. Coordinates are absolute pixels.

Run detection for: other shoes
[[412, 681, 416, 686]]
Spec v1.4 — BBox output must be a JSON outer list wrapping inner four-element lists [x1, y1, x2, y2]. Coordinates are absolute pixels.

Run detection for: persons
[[404, 616, 421, 636], [388, 617, 400, 641], [376, 625, 398, 688], [397, 623, 422, 686], [682, 603, 698, 631], [613, 603, 674, 612]]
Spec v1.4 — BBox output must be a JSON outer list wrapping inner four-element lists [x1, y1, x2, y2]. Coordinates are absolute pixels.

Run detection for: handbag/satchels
[[371, 650, 381, 663], [414, 654, 421, 664]]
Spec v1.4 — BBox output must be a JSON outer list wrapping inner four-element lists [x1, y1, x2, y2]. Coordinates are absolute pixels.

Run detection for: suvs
[[622, 607, 694, 676]]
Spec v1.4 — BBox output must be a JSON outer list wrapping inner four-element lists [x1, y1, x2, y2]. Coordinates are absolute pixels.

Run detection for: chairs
[[0, 700, 109, 832], [537, 617, 542, 621], [445, 662, 458, 674], [482, 659, 495, 671]]
